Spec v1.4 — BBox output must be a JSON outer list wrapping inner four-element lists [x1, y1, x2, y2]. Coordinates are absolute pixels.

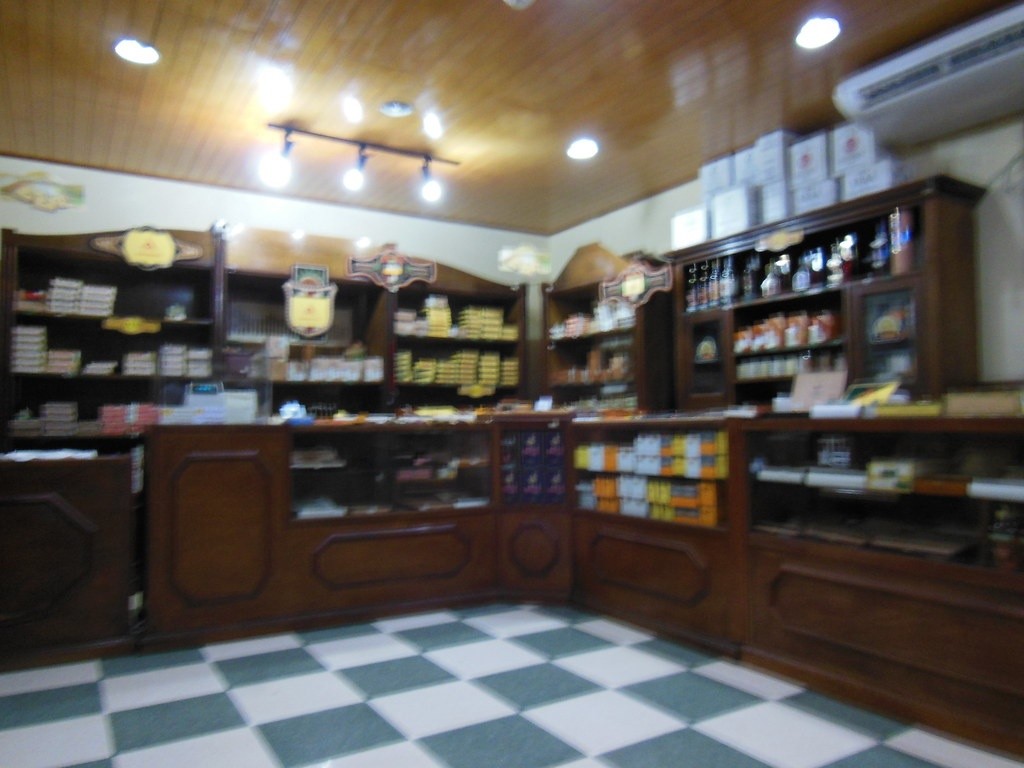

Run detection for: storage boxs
[[668, 125, 906, 251]]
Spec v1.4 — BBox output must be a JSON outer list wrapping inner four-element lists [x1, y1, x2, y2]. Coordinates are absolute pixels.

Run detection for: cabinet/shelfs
[[0, 174, 1024, 760]]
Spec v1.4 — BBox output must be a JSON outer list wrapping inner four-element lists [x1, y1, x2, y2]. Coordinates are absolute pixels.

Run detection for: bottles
[[991, 505, 1013, 560]]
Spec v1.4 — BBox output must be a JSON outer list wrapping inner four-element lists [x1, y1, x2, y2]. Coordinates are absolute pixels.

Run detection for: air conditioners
[[833, 0, 1024, 149]]
